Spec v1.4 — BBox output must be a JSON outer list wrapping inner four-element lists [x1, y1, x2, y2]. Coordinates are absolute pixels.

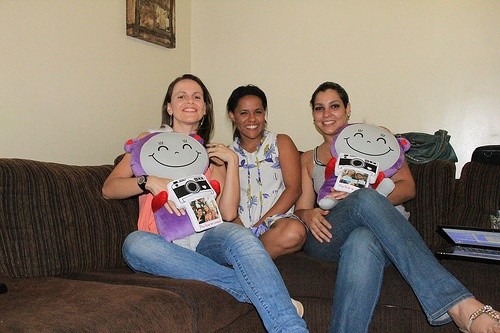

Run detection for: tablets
[[436, 225, 500, 250]]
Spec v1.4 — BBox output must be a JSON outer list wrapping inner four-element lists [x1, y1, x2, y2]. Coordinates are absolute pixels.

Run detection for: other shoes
[[290, 297, 305, 317]]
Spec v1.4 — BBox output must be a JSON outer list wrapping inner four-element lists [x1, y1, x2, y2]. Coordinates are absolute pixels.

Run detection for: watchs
[[136, 175, 150, 193]]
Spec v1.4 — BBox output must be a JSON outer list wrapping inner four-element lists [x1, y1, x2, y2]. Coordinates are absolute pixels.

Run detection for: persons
[[203, 204, 218, 221], [196, 208, 205, 223], [100, 72, 311, 333], [293, 81, 500, 333], [227, 84, 308, 261], [341, 169, 370, 189]]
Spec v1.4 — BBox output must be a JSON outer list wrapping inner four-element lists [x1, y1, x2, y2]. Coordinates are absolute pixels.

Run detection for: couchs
[[0, 144, 500, 333]]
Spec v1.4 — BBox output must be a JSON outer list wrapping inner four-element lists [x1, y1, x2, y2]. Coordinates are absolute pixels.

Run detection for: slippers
[[456, 305, 500, 333]]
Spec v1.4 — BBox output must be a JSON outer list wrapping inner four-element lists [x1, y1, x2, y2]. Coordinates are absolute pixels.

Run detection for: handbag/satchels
[[392, 129, 458, 165]]
